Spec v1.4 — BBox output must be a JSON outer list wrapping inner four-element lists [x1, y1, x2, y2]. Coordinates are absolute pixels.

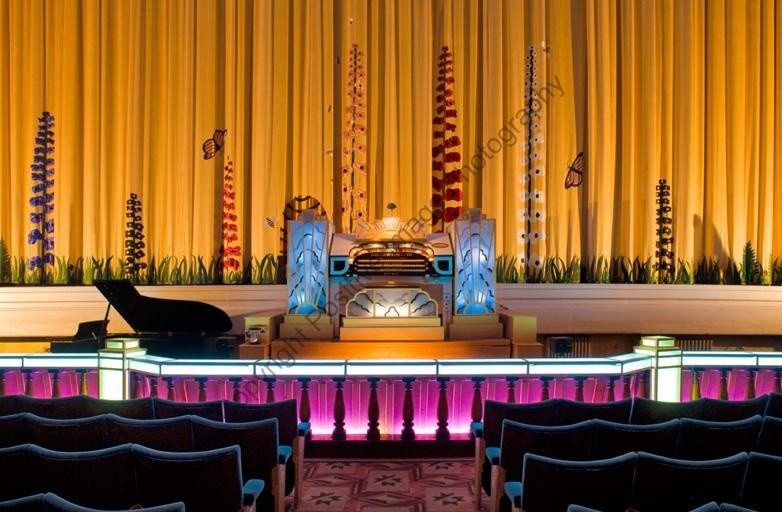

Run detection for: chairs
[[26, 413, 106, 449], [42, 492, 185, 512], [700, 394, 769, 421], [153, 398, 223, 423], [471, 398, 559, 505], [675, 415, 763, 459], [0, 493, 42, 512], [0, 395, 153, 419], [31, 442, 132, 492], [633, 451, 749, 512], [559, 397, 633, 425], [720, 504, 755, 512], [630, 398, 705, 426], [566, 500, 720, 512], [592, 418, 681, 459], [224, 399, 311, 506], [765, 391, 782, 418], [481, 418, 592, 512], [0, 413, 26, 445], [106, 413, 190, 451], [190, 415, 296, 512], [756, 416, 782, 455], [741, 451, 782, 512], [504, 452, 637, 512], [0, 443, 31, 495], [132, 444, 265, 512]]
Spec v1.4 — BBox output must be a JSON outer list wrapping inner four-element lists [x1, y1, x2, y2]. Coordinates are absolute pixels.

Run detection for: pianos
[[91, 277, 234, 357]]
